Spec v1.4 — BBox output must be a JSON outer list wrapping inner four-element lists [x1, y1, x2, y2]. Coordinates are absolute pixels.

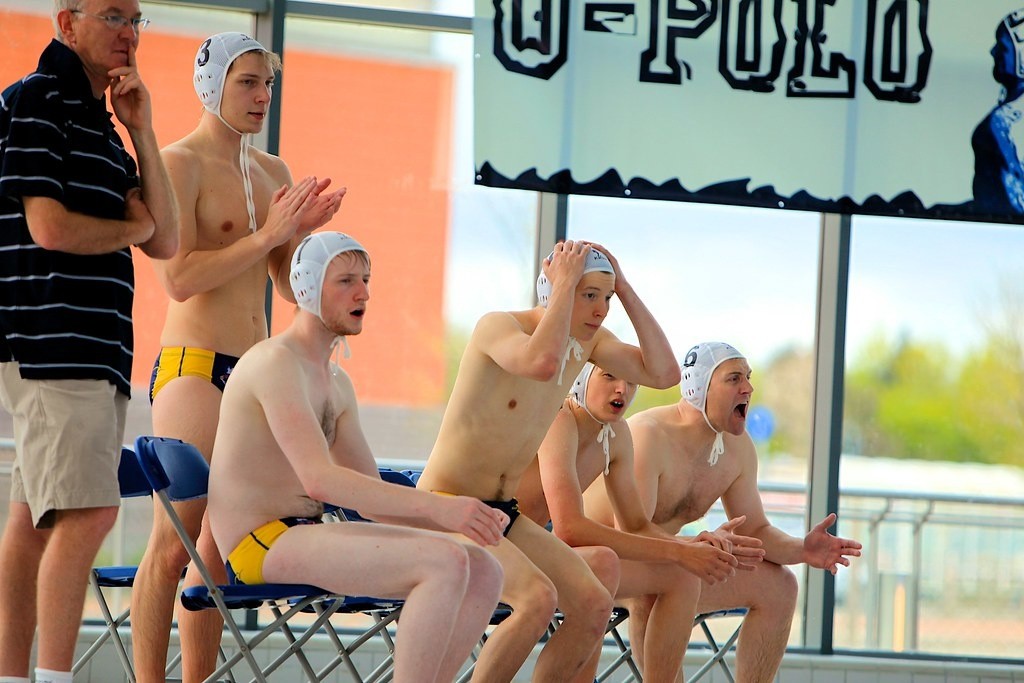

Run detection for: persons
[[415, 238, 684, 683], [514, 361, 737, 682], [580, 340, 861, 683], [207, 232, 512, 682], [0, 0, 177, 683], [127, 30, 344, 682]]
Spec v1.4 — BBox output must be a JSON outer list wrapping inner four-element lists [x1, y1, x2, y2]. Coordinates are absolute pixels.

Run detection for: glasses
[[69, 8, 151, 33]]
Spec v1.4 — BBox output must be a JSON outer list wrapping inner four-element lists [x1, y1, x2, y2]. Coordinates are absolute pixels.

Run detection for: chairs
[[69, 436, 748, 683]]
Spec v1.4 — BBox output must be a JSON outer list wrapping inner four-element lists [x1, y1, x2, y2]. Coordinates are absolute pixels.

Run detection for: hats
[[536, 243, 616, 309], [290, 231, 369, 317], [194, 32, 268, 115], [681, 341, 759, 411], [570, 362, 639, 409]]
